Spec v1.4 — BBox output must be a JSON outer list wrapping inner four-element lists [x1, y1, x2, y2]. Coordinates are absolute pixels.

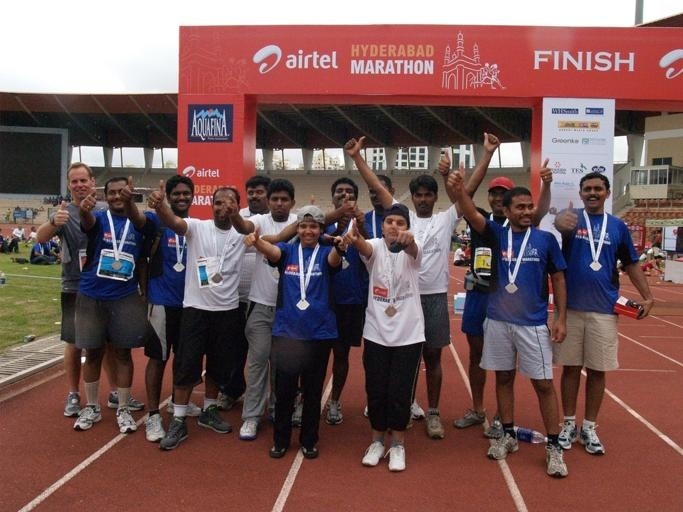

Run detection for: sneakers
[[483, 416, 503, 439], [558, 421, 578, 449], [267, 400, 343, 459], [580, 425, 605, 455], [407, 399, 445, 439], [362, 441, 385, 466], [545, 445, 568, 477], [487, 433, 519, 460], [240, 416, 262, 441], [384, 445, 406, 471], [63, 393, 238, 450], [454, 409, 485, 428]]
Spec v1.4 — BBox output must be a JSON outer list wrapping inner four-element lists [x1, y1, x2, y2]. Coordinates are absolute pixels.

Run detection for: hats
[[296, 205, 325, 226], [488, 177, 515, 192], [384, 204, 409, 218]]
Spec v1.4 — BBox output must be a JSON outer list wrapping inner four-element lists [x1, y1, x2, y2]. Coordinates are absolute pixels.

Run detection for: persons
[[35, 132, 498, 471], [0, 206, 62, 265], [438, 150, 553, 437], [446, 158, 568, 478], [637, 230, 665, 275], [534, 172, 655, 455], [452, 227, 471, 267]]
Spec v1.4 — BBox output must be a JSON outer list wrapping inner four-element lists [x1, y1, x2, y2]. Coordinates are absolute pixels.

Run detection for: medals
[[589, 261, 602, 271], [505, 283, 518, 294]]
